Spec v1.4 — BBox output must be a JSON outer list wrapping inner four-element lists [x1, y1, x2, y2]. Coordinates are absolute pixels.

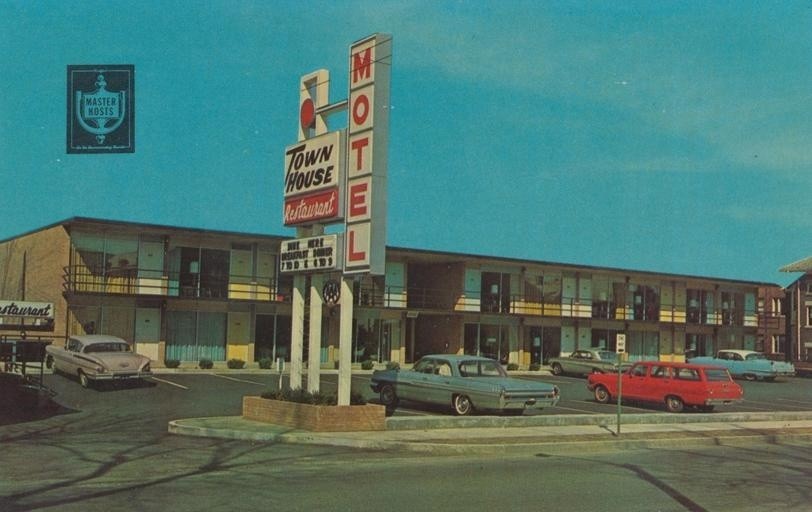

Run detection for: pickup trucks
[[687, 349, 796, 382], [46, 334, 153, 387]]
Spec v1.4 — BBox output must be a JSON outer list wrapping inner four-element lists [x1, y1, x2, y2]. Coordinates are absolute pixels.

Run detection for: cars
[[370, 354, 561, 415], [548, 347, 635, 378], [584, 360, 744, 412]]
